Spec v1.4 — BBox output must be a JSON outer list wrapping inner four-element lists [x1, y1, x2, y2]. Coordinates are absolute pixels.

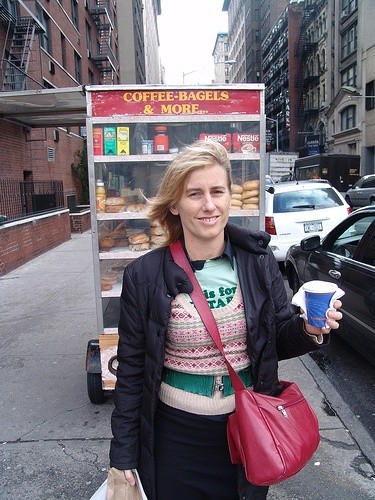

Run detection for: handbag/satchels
[[227, 382, 319, 485]]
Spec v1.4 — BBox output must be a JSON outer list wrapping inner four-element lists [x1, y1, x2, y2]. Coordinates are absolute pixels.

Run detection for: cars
[[266, 179, 352, 276]]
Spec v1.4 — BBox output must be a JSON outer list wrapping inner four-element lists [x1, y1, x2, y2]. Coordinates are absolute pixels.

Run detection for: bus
[[290, 153, 362, 190]]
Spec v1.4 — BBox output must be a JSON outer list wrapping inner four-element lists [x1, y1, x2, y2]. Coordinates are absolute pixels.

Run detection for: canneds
[[153, 126, 169, 153]]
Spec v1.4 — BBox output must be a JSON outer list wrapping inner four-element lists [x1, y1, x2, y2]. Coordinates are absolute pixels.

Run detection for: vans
[[266, 175, 274, 184], [283, 203, 375, 368], [345, 174, 375, 208]]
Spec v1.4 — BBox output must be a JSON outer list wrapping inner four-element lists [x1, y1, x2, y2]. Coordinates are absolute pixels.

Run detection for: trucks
[[267, 152, 300, 183]]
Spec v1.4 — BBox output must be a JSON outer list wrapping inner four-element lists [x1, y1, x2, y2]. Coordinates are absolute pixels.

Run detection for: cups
[[303, 280, 338, 328]]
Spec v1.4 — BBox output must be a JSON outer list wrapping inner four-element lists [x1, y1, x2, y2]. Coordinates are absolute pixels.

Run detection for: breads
[[101, 267, 124, 291], [106, 197, 146, 213], [229, 179, 259, 210], [99, 218, 169, 252]]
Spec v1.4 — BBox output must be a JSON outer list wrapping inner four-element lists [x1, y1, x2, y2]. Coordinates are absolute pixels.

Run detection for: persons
[[108, 140, 344, 500]]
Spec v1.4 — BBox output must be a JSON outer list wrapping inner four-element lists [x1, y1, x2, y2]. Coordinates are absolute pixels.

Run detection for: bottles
[[96, 183, 106, 212]]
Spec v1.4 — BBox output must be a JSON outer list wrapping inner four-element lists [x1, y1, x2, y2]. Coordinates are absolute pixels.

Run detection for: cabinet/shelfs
[[85, 83, 265, 390]]
